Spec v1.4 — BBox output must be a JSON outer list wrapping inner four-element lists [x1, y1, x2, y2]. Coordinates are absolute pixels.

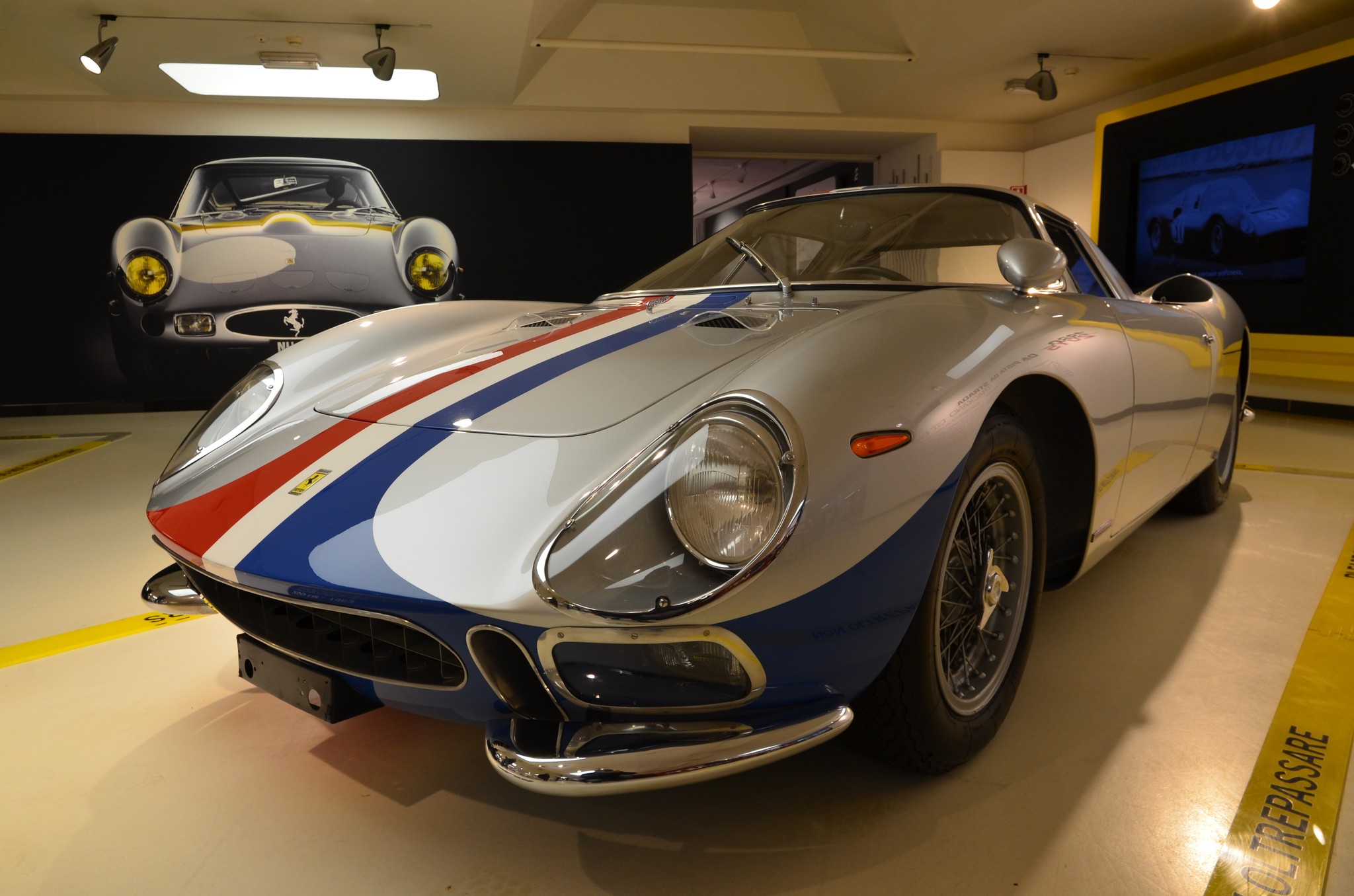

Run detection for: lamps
[[360, 26, 397, 80], [691, 160, 751, 207], [1026, 50, 1061, 102], [79, 12, 132, 78]]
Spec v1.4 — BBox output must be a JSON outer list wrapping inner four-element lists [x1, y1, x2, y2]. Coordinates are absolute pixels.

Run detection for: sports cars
[[1140, 176, 1309, 261], [136, 184, 1256, 799], [109, 153, 462, 363]]
